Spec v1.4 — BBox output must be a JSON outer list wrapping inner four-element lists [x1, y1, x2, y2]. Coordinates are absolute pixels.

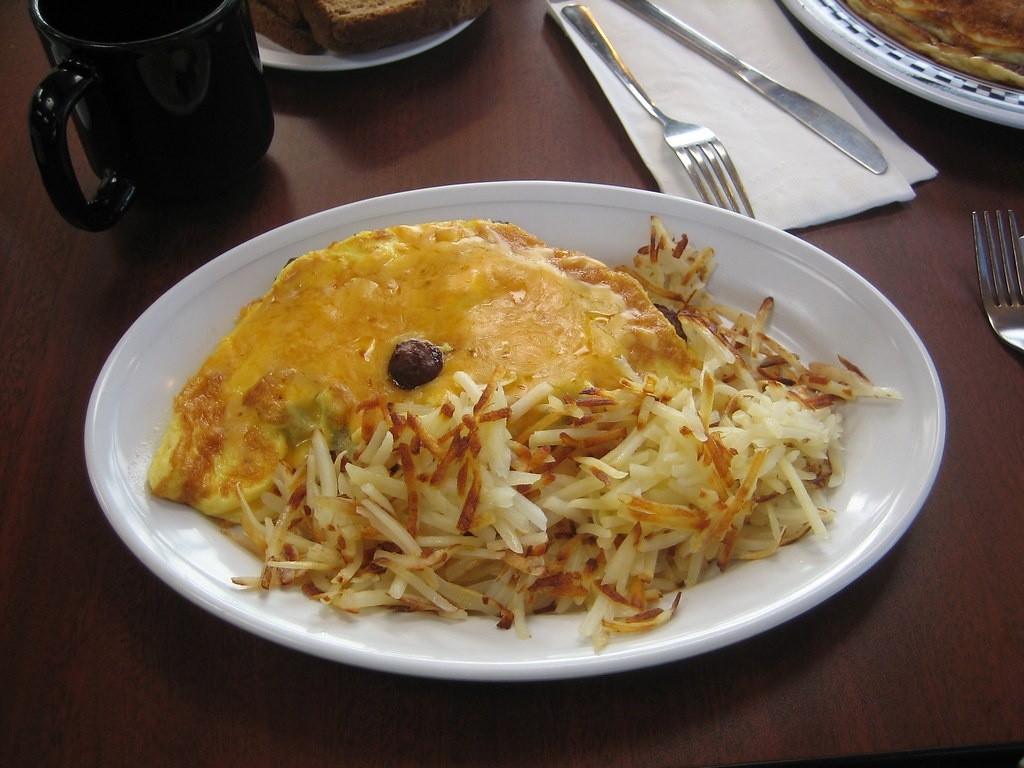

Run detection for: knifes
[[609, 0, 887, 176]]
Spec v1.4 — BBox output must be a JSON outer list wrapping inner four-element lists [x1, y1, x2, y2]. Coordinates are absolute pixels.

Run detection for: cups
[[24, 0, 276, 232]]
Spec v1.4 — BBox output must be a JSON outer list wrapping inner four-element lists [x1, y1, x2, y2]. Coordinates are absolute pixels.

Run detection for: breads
[[247, 0, 483, 53]]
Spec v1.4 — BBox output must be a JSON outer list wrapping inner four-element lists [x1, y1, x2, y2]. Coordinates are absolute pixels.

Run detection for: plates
[[778, 0, 1024, 130], [252, 0, 494, 91], [83, 178, 946, 682]]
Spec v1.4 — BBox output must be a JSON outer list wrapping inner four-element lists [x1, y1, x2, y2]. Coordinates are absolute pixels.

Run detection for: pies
[[847, 0, 1024, 91]]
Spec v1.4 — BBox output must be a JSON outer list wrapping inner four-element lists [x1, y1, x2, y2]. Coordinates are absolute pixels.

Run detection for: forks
[[971, 208, 1024, 351], [562, 4, 756, 221]]
